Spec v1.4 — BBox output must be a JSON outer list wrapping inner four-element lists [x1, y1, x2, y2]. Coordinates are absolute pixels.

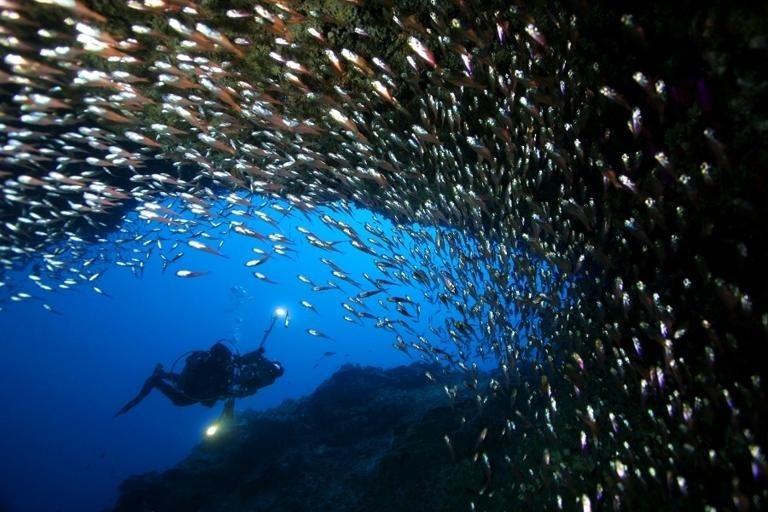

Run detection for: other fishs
[[0, 0, 767, 512]]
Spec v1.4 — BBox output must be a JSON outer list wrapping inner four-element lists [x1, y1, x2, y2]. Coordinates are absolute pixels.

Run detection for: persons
[[113, 343, 284, 419]]
